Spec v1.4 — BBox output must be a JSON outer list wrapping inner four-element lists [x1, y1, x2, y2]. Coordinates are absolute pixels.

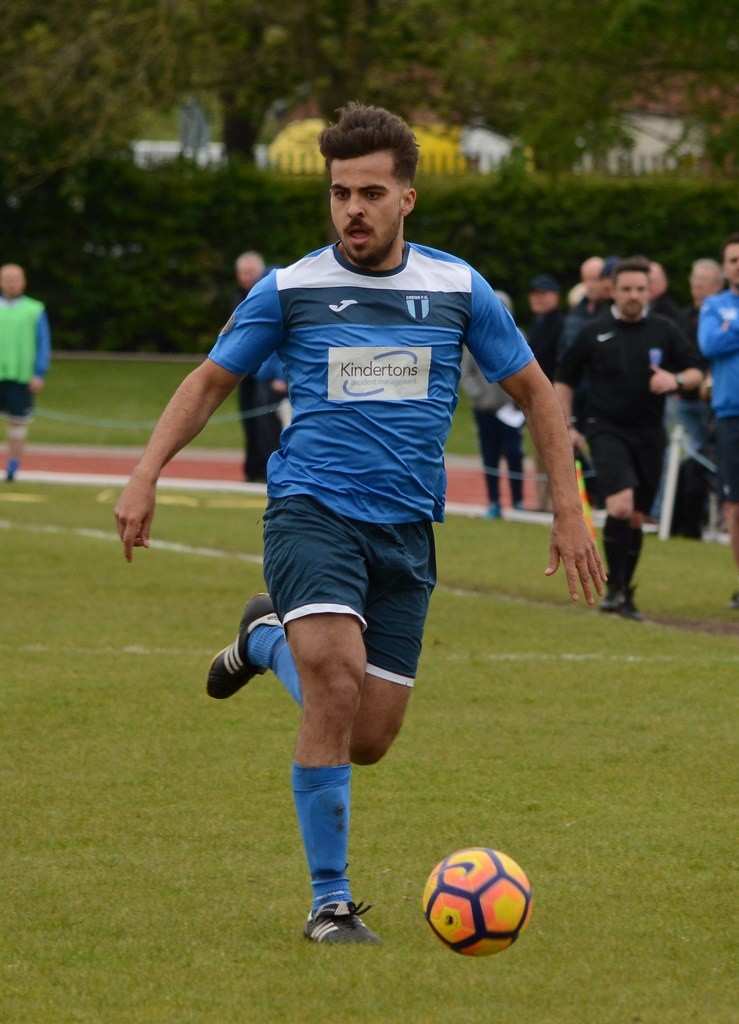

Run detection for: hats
[[527, 277, 560, 292], [600, 258, 620, 279]]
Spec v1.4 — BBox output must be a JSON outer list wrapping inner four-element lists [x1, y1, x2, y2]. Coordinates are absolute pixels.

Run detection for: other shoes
[[512, 502, 524, 510], [5, 471, 15, 482], [727, 591, 739, 609], [620, 585, 643, 622], [485, 503, 500, 519], [599, 598, 619, 612]]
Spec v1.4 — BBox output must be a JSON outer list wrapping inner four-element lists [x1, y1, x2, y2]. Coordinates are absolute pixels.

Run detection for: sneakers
[[206, 592, 281, 699], [303, 894, 385, 948]]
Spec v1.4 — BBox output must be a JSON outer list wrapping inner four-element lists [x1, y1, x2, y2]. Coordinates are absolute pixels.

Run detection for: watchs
[[675, 371, 684, 389]]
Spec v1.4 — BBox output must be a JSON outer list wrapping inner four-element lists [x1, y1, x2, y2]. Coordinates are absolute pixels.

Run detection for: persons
[[553, 256, 708, 621], [696, 233, 738, 611], [209, 250, 290, 485], [461, 290, 528, 519], [0, 264, 52, 482], [525, 257, 729, 545], [114, 101, 607, 946]]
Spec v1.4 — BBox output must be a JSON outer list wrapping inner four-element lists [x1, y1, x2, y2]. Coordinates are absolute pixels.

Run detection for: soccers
[[421, 843, 535, 959]]
